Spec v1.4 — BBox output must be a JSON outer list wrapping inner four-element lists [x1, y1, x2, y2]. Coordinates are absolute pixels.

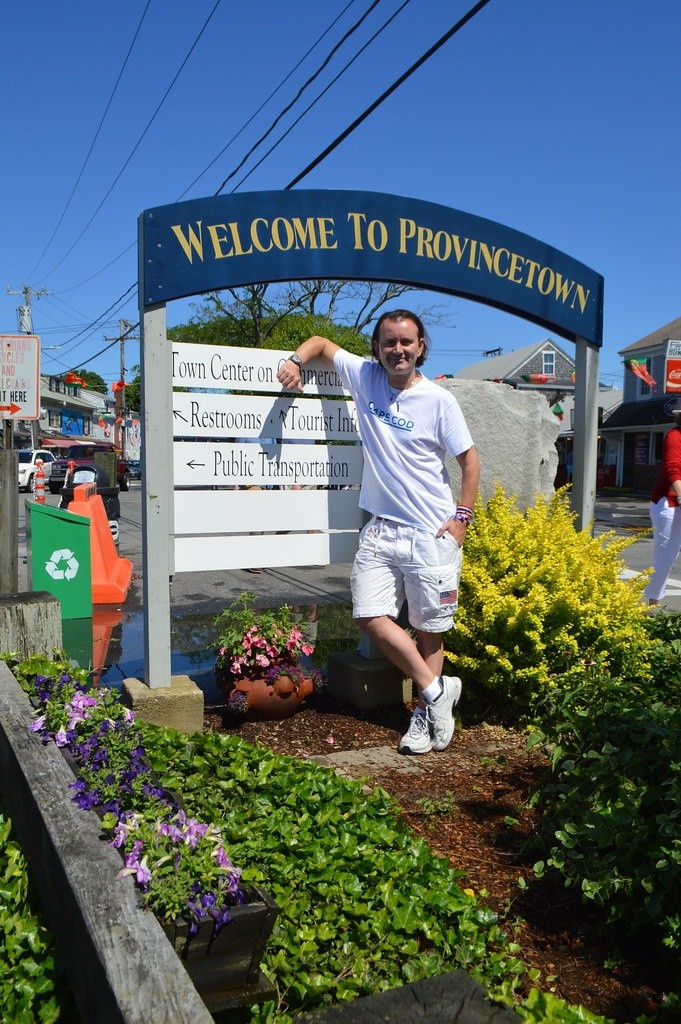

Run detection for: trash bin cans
[[58, 464, 121, 557], [25, 499, 93, 621]]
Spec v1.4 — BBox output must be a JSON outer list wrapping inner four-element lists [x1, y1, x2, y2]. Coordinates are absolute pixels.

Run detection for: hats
[[672, 398, 681, 414]]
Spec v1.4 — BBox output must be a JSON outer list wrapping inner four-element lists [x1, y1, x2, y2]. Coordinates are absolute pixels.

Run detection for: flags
[[622, 355, 657, 386]]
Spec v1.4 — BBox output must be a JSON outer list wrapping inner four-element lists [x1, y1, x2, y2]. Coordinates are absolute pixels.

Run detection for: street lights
[[40, 345, 62, 349]]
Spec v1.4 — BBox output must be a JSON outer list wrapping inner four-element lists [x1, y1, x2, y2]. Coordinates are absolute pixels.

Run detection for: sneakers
[[423, 675, 462, 750], [398, 713, 432, 755]]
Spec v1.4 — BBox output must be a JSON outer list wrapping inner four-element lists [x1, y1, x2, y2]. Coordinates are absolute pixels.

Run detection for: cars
[[15, 449, 57, 493], [129, 460, 142, 480]]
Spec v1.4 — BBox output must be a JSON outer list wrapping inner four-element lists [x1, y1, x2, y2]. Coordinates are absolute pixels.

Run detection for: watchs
[[287, 354, 302, 368]]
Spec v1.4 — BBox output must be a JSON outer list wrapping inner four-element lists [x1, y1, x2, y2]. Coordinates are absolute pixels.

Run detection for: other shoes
[[649, 608, 671, 615]]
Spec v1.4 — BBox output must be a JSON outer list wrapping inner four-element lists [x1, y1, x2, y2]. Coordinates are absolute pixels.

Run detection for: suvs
[[48, 442, 130, 494]]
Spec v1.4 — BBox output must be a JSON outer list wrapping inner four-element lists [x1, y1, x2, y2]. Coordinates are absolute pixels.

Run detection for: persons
[[644, 404, 681, 609], [278, 310, 480, 753]]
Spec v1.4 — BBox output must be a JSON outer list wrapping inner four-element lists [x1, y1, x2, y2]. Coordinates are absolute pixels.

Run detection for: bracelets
[[455, 502, 474, 525]]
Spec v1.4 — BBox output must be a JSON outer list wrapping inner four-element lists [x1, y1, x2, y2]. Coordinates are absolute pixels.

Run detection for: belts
[[376, 517, 392, 522]]
